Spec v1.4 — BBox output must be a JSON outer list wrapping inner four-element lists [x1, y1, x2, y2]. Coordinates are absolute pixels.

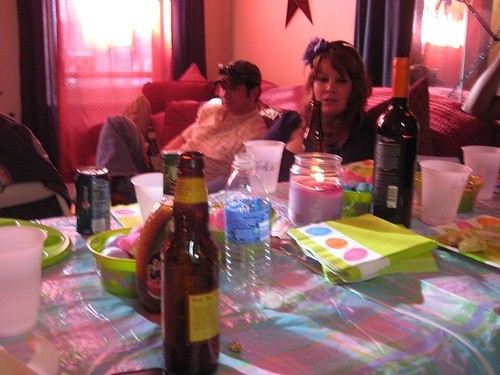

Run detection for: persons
[[94, 61, 269, 208], [1, 113, 74, 220], [205, 39, 379, 199]]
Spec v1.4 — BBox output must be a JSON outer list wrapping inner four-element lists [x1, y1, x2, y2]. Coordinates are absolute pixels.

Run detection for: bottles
[[223, 154, 272, 301], [288, 151, 344, 228], [374, 58, 421, 228], [136, 153, 181, 314], [160, 151, 221, 374]]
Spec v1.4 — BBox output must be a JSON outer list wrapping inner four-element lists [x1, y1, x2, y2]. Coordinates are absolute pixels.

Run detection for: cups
[[131, 172, 163, 225], [242, 140, 285, 193], [419, 159, 473, 225], [461, 146, 500, 198], [0, 225, 47, 337]]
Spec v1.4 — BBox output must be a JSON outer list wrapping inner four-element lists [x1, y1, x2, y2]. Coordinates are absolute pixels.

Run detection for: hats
[[212, 59, 262, 89]]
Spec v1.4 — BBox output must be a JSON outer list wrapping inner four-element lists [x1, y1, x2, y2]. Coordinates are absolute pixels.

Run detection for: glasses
[[216, 61, 260, 76]]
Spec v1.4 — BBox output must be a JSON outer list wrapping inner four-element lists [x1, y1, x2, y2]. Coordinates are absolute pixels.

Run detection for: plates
[[414, 214, 500, 270]]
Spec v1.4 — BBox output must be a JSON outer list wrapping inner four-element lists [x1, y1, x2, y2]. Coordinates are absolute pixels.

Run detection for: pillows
[[164, 99, 200, 144], [177, 62, 206, 81], [143, 81, 212, 112]]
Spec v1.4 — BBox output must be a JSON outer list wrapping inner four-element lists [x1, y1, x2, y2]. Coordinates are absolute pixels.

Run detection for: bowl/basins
[[207, 203, 276, 259], [415, 170, 485, 213], [86, 227, 140, 297], [343, 165, 374, 217]]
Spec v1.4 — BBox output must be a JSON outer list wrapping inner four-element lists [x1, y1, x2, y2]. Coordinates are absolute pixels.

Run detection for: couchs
[[68, 76, 483, 148]]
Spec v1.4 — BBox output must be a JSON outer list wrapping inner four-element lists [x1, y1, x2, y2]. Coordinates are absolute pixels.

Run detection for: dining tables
[[0, 150, 500, 375]]
